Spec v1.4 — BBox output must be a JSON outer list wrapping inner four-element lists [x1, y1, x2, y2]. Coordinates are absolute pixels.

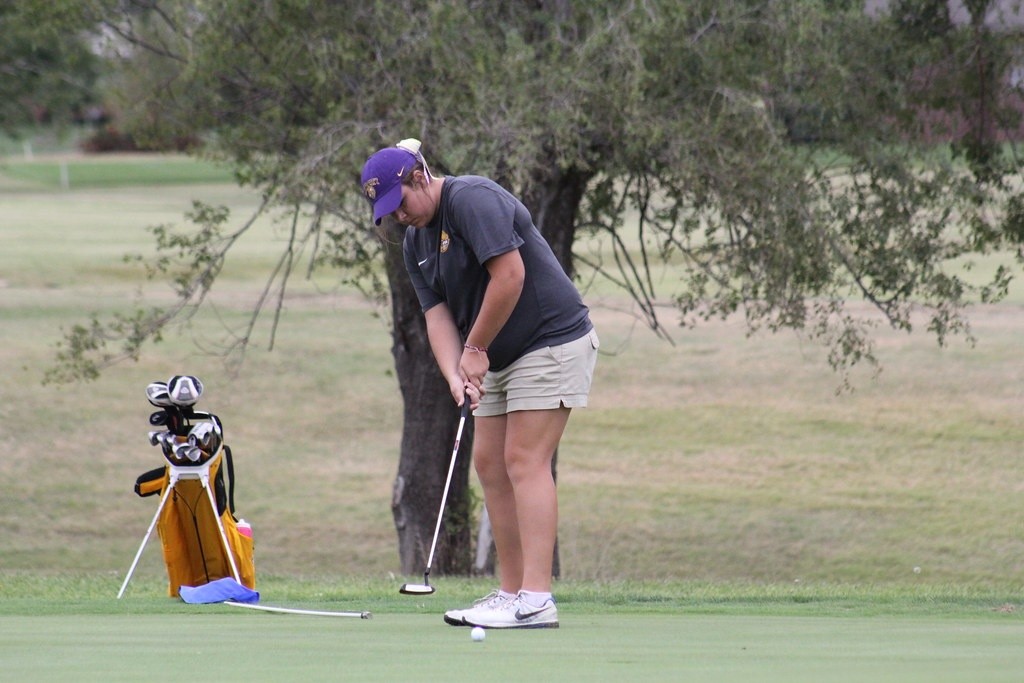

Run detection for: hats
[[361, 149, 421, 227]]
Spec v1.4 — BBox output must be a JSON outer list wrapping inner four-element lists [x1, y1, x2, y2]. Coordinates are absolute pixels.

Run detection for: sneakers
[[443, 589, 511, 626], [462, 592, 560, 629]]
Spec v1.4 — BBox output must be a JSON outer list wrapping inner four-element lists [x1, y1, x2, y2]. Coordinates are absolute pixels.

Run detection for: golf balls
[[471, 627, 486, 641]]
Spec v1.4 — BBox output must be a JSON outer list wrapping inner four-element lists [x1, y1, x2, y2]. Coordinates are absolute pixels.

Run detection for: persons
[[360, 142, 597, 630]]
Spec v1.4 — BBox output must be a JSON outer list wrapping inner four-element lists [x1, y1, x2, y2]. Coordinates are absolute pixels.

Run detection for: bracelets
[[465, 345, 488, 352]]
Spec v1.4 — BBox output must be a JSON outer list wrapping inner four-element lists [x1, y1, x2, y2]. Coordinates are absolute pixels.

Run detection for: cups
[[234, 519, 252, 539]]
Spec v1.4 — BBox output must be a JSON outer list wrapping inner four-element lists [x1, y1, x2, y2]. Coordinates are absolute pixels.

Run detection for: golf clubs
[[399, 394, 471, 595], [145, 376, 221, 463]]
